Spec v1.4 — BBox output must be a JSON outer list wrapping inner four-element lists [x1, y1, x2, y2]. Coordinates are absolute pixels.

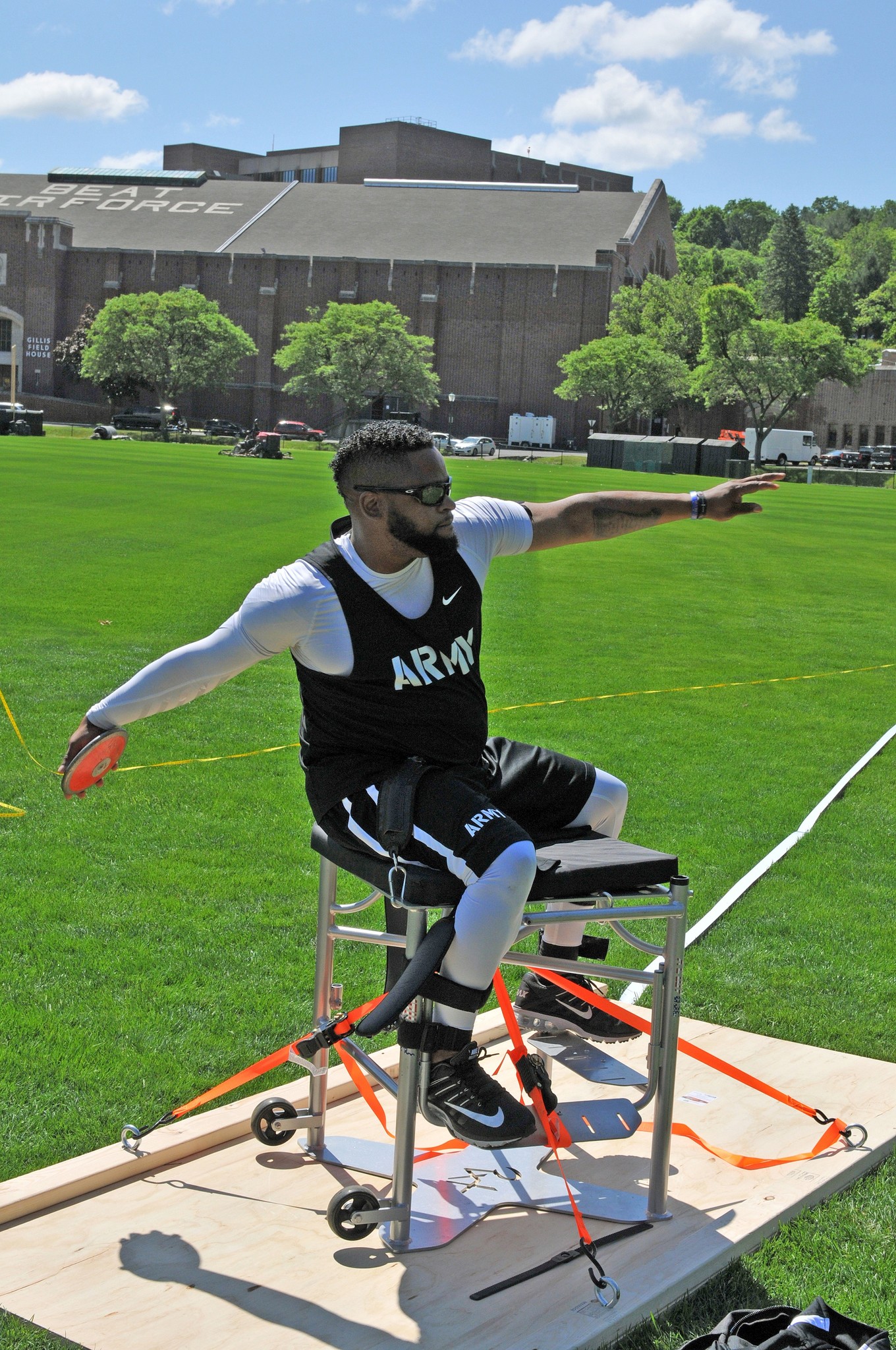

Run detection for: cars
[[0, 401, 26, 411], [110, 406, 187, 430], [429, 431, 463, 449], [819, 449, 855, 467], [452, 435, 496, 457]]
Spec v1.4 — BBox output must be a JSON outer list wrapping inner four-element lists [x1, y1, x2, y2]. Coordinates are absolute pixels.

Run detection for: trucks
[[744, 427, 821, 467]]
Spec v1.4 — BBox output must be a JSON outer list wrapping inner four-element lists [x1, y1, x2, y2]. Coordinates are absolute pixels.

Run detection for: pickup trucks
[[840, 444, 875, 469]]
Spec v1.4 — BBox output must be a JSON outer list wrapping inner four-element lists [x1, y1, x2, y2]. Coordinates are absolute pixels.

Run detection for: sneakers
[[429, 1041, 538, 1150], [515, 970, 643, 1043]]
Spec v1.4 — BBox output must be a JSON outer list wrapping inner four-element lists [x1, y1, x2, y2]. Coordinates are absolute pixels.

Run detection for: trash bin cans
[[724, 459, 752, 480]]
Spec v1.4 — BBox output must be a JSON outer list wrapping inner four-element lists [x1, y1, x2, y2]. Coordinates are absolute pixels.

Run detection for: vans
[[869, 445, 896, 471]]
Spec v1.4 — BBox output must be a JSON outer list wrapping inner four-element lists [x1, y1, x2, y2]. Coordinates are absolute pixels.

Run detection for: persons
[[56, 420, 787, 1150]]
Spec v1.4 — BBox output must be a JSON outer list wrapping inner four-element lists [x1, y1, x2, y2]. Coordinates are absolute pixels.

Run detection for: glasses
[[351, 477, 455, 507]]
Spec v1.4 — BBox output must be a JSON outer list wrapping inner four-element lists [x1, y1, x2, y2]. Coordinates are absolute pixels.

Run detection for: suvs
[[204, 418, 249, 438], [272, 420, 328, 442]]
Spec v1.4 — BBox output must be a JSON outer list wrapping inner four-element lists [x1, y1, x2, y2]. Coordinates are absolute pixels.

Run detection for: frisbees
[[61, 728, 127, 796]]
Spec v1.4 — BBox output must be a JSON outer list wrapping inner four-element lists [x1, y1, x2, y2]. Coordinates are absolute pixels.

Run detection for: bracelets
[[689, 491, 707, 520]]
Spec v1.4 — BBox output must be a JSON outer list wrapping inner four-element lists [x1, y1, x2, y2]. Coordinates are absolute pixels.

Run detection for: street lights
[[444, 393, 456, 455]]
